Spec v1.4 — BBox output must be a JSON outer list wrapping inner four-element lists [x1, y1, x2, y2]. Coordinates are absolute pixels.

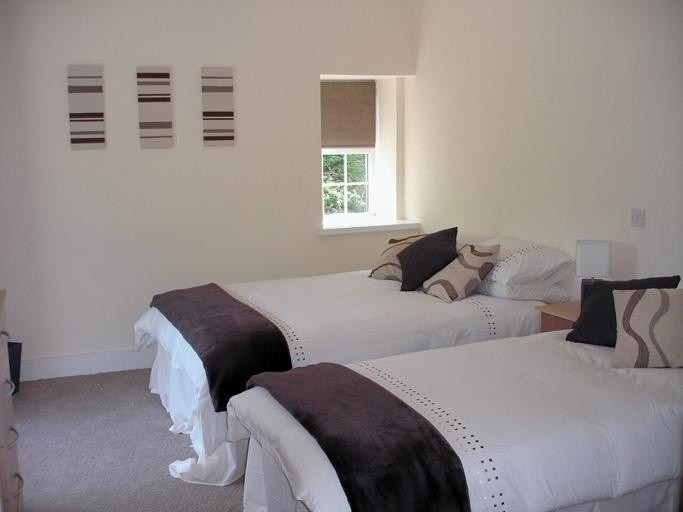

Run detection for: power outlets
[[629, 208, 645, 228]]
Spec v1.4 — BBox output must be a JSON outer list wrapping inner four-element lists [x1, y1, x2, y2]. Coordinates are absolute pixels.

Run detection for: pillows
[[476, 233, 576, 289], [418, 243, 500, 303], [472, 279, 571, 304], [398, 228, 458, 292], [565, 274, 681, 346], [613, 289, 682, 369], [368, 232, 431, 281]]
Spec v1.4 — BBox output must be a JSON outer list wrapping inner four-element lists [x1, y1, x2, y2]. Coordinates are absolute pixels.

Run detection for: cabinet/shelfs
[[0, 288, 27, 512]]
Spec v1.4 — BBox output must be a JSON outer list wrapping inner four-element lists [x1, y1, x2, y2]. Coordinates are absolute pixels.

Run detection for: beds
[[133, 271, 549, 487], [224, 326, 682, 512]]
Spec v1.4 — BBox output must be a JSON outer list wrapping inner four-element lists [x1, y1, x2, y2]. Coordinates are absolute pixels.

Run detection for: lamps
[[574, 239, 612, 279]]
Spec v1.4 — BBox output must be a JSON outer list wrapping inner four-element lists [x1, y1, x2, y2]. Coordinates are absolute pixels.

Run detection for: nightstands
[[534, 300, 581, 332]]
